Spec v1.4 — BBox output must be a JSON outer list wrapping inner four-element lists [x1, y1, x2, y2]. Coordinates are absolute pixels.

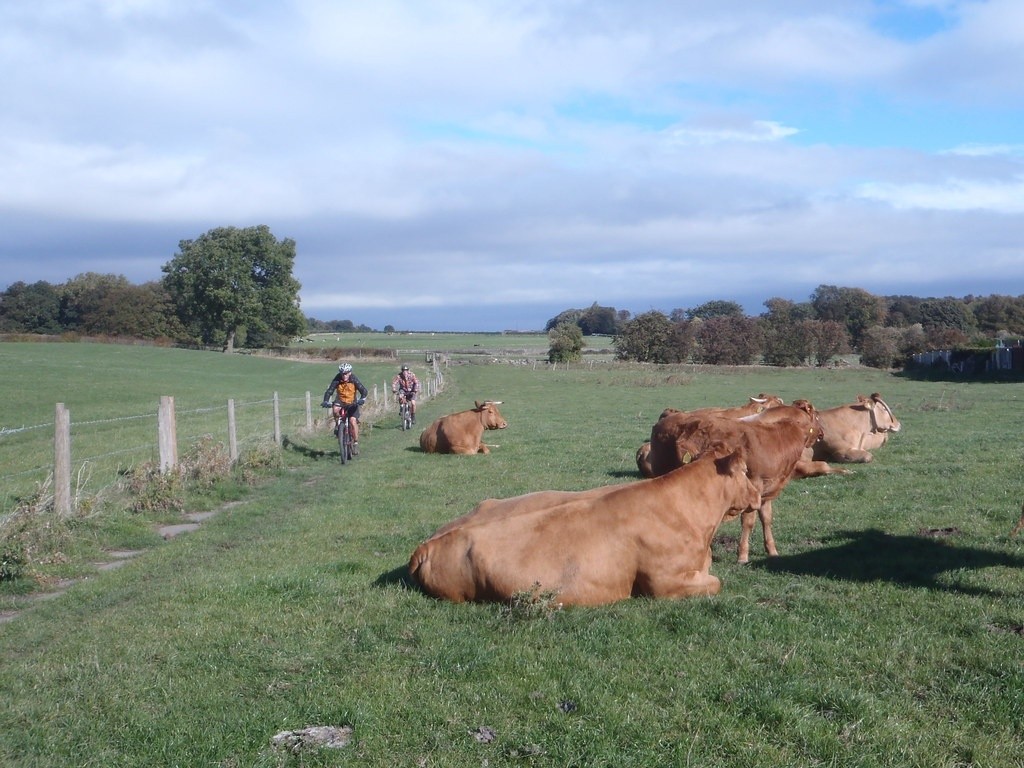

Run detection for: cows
[[635, 392, 858, 522], [814, 390, 902, 464], [419, 400, 508, 454], [406, 437, 763, 608], [648, 399, 826, 564]]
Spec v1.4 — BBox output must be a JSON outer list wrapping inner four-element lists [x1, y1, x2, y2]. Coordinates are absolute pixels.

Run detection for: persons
[[322, 363, 368, 455], [391, 364, 418, 424]]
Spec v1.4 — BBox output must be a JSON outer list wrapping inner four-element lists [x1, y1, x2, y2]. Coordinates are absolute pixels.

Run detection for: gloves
[[410, 392, 416, 396], [321, 402, 329, 408], [357, 400, 364, 405], [393, 391, 399, 395]]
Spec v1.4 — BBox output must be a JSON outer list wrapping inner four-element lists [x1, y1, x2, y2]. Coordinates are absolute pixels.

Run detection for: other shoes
[[411, 416, 416, 423], [353, 445, 359, 454], [334, 428, 338, 434], [399, 411, 402, 415]]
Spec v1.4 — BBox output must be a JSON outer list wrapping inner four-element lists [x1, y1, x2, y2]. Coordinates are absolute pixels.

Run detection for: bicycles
[[394, 390, 414, 431], [323, 402, 357, 464]]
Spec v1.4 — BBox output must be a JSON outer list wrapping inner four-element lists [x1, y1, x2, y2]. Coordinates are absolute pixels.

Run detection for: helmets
[[338, 363, 352, 374], [401, 365, 409, 371]]
